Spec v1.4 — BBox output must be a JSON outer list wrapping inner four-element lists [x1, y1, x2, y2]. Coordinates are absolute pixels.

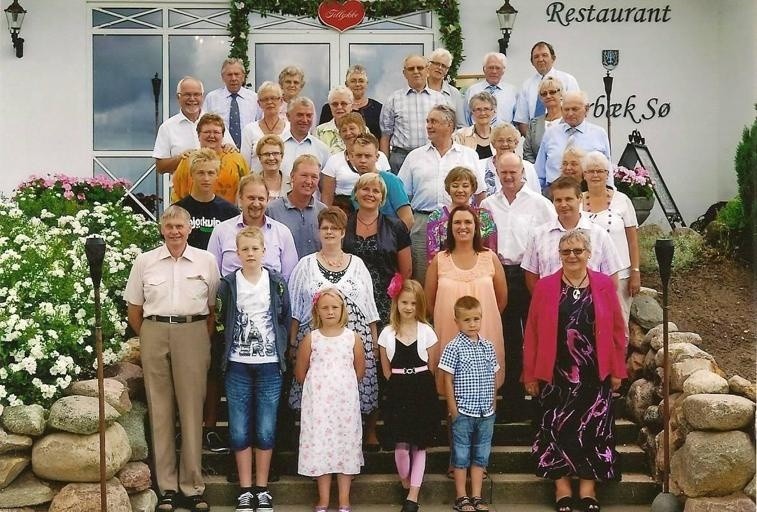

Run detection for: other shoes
[[268, 460, 280, 482], [366, 443, 382, 453], [226, 466, 239, 483], [497, 417, 536, 446], [314, 505, 328, 511], [338, 506, 352, 511]]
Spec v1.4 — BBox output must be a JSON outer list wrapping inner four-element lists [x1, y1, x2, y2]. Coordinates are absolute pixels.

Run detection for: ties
[[559, 128, 576, 178], [228, 93, 241, 151], [488, 86, 497, 129], [534, 94, 545, 119]]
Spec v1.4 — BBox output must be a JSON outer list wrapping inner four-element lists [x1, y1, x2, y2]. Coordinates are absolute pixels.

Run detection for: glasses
[[256, 152, 282, 156], [403, 66, 426, 72], [539, 89, 560, 97]]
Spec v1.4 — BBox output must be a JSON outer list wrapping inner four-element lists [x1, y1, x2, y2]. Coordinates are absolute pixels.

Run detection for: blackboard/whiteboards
[[633, 143, 680, 216]]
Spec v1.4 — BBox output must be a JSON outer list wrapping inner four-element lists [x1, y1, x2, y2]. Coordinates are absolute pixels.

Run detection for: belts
[[145, 314, 209, 324]]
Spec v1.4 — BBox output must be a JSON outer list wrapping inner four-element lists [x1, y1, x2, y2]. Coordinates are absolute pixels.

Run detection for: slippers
[[578, 496, 599, 511], [556, 496, 576, 511]]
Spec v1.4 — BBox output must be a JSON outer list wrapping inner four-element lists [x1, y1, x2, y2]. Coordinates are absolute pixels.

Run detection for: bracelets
[[632, 268, 639, 271]]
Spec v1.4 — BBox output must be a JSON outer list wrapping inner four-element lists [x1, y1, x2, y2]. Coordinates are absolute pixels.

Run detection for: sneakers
[[254, 490, 274, 511], [401, 500, 419, 511], [235, 491, 254, 511], [174, 427, 182, 452], [400, 481, 410, 504], [203, 426, 230, 452]]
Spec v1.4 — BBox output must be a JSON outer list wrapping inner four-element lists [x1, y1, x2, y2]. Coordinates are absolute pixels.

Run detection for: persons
[[437, 296, 500, 512], [579, 151, 642, 398], [218, 227, 292, 512], [151, 41, 616, 479], [122, 206, 221, 512], [295, 289, 367, 512], [376, 279, 438, 512], [523, 232, 626, 512]]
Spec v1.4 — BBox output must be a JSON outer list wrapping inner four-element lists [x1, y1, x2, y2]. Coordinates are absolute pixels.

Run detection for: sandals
[[453, 496, 476, 511], [177, 492, 210, 511], [447, 471, 454, 479], [156, 490, 177, 511], [483, 471, 488, 479], [469, 496, 489, 511]]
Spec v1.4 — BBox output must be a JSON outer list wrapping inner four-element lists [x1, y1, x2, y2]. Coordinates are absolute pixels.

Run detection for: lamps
[[495, 0, 520, 57], [3, 1, 30, 60]]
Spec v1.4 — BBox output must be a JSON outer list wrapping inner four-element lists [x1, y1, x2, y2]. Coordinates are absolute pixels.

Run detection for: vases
[[630, 198, 657, 226]]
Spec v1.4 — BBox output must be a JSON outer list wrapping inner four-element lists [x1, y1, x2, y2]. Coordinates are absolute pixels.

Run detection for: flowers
[[613, 163, 657, 199]]
